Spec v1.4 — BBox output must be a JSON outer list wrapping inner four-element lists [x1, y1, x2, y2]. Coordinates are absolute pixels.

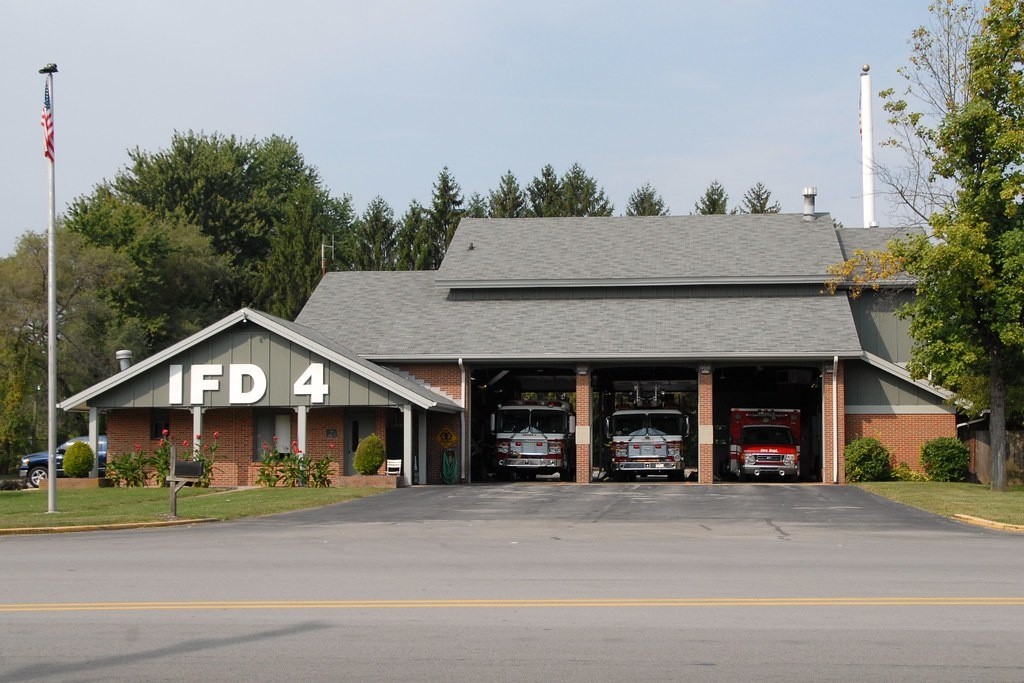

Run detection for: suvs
[[19, 435, 108, 488]]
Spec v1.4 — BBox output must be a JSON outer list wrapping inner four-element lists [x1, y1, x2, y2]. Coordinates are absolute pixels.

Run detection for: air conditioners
[[777, 370, 812, 384]]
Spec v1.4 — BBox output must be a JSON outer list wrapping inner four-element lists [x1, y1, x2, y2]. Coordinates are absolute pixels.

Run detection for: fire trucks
[[605, 408, 691, 482], [725, 407, 802, 483], [489, 399, 576, 481]]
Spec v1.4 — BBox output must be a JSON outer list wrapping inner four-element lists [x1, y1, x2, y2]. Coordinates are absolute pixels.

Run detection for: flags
[[40, 78, 55, 162]]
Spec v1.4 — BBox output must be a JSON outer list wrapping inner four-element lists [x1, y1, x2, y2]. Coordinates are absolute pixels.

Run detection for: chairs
[[384, 459, 402, 476]]
